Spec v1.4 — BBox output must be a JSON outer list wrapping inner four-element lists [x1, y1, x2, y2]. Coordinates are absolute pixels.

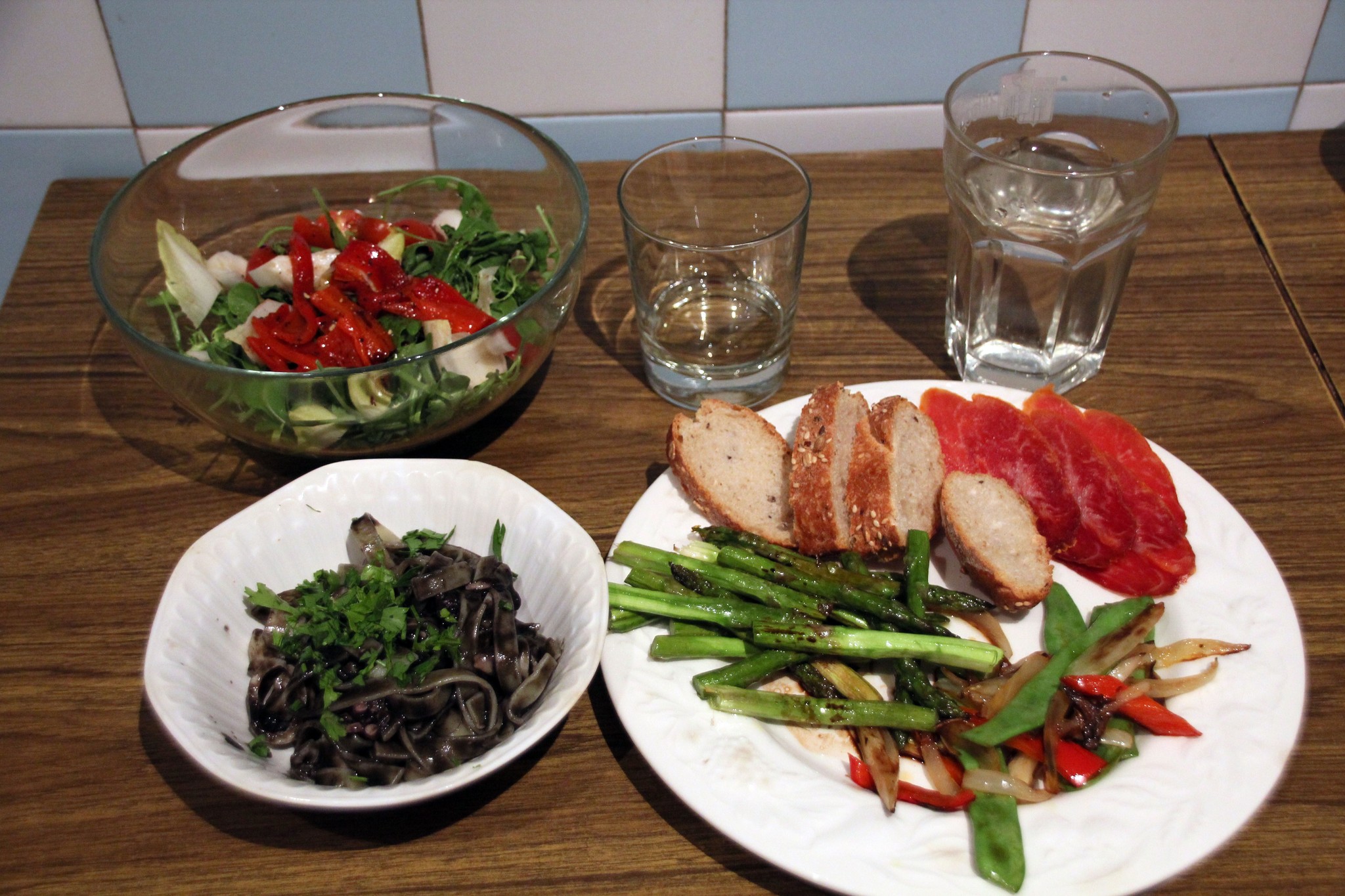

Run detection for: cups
[[616, 136, 810, 415], [939, 50, 1180, 410]]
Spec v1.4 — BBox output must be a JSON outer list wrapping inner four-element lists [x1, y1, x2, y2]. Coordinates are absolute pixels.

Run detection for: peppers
[[245, 208, 536, 374]]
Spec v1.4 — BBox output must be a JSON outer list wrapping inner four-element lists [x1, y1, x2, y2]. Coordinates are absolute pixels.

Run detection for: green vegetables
[[143, 174, 563, 454]]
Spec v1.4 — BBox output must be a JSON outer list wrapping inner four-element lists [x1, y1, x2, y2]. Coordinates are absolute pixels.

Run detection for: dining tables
[[0, 135, 1343, 896]]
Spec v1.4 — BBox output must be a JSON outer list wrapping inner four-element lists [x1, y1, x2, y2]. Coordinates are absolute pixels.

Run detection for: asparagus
[[607, 522, 1005, 753]]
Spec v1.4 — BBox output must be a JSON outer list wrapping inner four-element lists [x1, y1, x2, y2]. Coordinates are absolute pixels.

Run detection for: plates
[[601, 370, 1310, 895]]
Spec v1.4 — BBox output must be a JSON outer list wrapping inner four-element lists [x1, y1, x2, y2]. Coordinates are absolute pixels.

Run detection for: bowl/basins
[[144, 450, 610, 813], [89, 94, 591, 453]]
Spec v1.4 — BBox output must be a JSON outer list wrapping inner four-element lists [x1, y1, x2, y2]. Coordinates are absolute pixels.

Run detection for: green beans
[[953, 580, 1156, 892]]
